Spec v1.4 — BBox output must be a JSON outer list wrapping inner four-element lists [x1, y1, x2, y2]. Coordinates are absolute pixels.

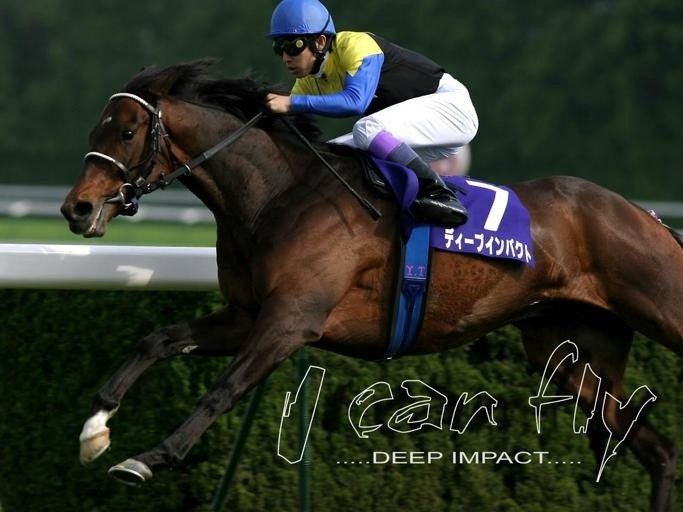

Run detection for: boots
[[386, 142, 469, 227]]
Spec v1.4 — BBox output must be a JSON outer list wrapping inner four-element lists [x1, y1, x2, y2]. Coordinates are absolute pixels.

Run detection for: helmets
[[264, 0, 337, 38]]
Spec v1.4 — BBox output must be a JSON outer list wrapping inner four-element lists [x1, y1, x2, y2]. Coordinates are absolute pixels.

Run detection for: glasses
[[272, 35, 315, 57]]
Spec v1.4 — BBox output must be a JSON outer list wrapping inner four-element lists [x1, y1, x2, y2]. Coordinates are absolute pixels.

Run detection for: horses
[[59, 54, 683, 511]]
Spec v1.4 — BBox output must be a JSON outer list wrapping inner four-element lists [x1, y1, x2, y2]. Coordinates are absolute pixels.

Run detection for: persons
[[265, 0, 479, 228]]
